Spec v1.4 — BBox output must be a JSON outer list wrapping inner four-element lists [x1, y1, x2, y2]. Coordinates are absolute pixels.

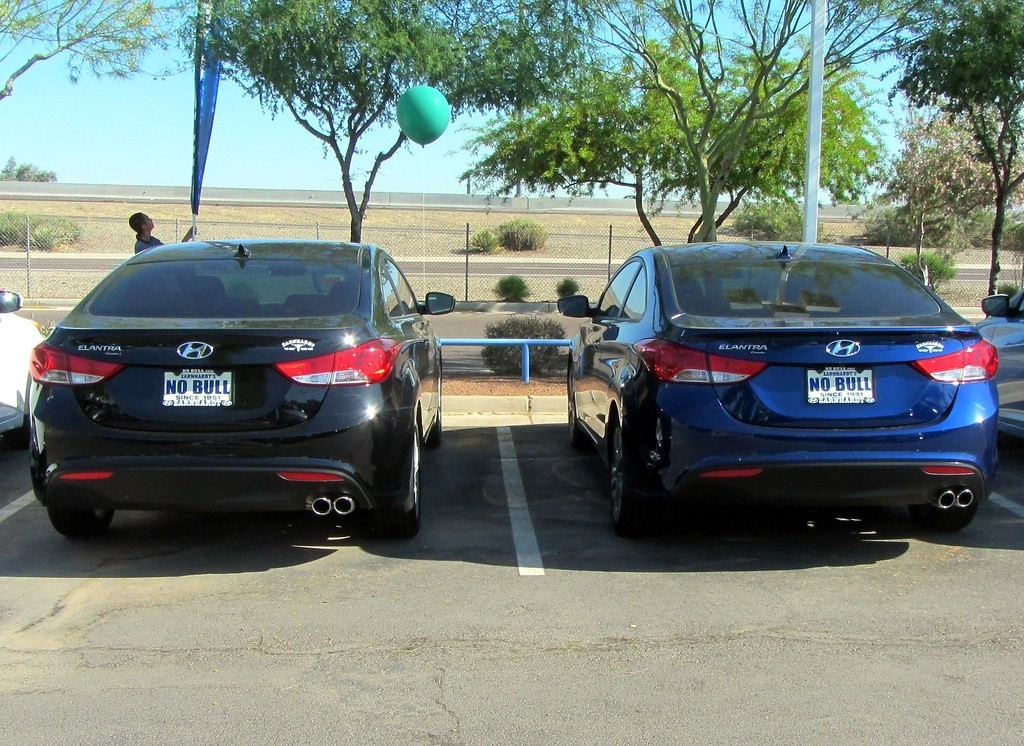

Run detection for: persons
[[129, 213, 199, 254]]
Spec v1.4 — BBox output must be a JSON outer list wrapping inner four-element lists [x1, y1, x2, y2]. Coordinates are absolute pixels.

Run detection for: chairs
[[675, 278, 732, 313], [284, 282, 354, 317], [152, 276, 231, 319]]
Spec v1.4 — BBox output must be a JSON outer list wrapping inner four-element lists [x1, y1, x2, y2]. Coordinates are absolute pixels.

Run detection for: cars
[[558, 242, 1000, 545], [976, 286, 1024, 440], [21, 237, 456, 541], [0, 291, 47, 445]]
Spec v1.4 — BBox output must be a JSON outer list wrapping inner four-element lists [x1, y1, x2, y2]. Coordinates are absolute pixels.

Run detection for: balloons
[[398, 85, 451, 145]]
